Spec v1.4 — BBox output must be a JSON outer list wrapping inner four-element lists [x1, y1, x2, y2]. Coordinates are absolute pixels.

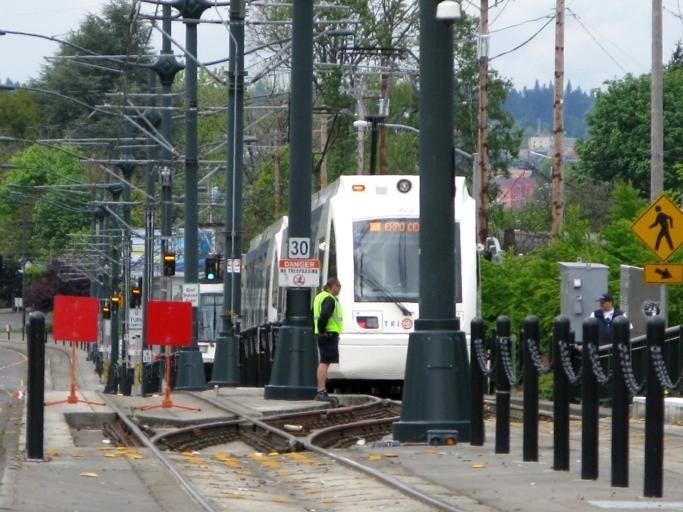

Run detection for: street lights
[[153, 30, 353, 392], [353, 120, 482, 319], [0, 29, 178, 394]]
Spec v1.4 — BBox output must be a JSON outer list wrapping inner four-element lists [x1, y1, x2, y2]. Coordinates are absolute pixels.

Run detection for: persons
[[312, 276, 343, 401], [589, 294, 633, 346]]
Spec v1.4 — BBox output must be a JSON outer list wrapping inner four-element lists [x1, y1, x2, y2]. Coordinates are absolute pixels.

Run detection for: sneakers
[[315, 389, 336, 401]]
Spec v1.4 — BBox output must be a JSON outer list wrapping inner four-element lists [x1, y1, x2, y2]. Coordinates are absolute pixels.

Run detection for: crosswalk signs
[[630, 194, 683, 264]]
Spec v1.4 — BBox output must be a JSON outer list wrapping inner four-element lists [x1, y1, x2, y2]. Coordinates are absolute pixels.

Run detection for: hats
[[596, 294, 612, 301]]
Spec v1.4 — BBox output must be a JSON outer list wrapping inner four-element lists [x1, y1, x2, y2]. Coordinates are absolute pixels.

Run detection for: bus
[[174, 283, 224, 365], [241, 175, 478, 398]]
